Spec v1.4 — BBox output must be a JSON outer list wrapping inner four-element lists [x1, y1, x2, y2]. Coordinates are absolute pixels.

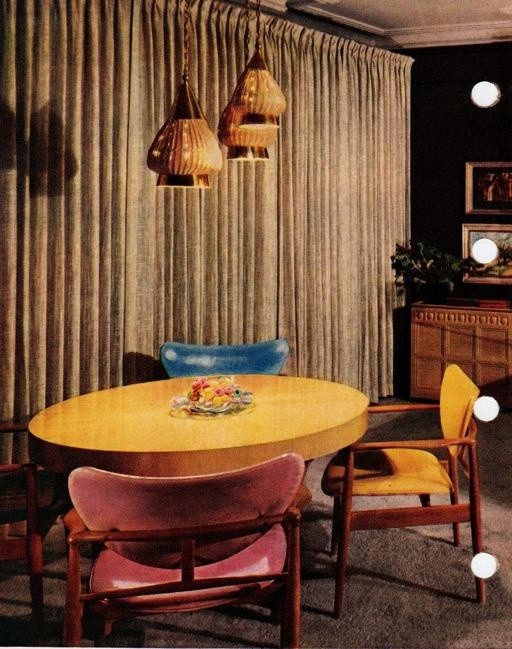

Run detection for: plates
[[170, 383, 252, 415]]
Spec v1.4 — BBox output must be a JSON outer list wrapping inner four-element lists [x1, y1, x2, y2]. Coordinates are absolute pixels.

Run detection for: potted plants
[[390, 240, 483, 306]]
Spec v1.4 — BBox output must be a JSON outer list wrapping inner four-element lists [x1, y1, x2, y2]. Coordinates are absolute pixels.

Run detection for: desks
[[31, 375, 367, 621]]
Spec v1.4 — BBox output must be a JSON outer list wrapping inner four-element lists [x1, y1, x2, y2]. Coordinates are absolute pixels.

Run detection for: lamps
[[147, 0, 285, 186]]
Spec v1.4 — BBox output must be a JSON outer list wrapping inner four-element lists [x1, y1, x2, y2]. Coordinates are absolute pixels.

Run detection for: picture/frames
[[462, 161, 512, 284]]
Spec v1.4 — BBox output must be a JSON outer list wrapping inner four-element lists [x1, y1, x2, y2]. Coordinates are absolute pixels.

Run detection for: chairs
[[63, 454, 312, 648], [320, 364, 484, 619], [1, 425, 70, 625], [159, 339, 315, 487]]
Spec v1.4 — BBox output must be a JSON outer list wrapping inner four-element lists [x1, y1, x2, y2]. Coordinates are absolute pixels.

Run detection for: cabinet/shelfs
[[410, 298, 512, 409]]
